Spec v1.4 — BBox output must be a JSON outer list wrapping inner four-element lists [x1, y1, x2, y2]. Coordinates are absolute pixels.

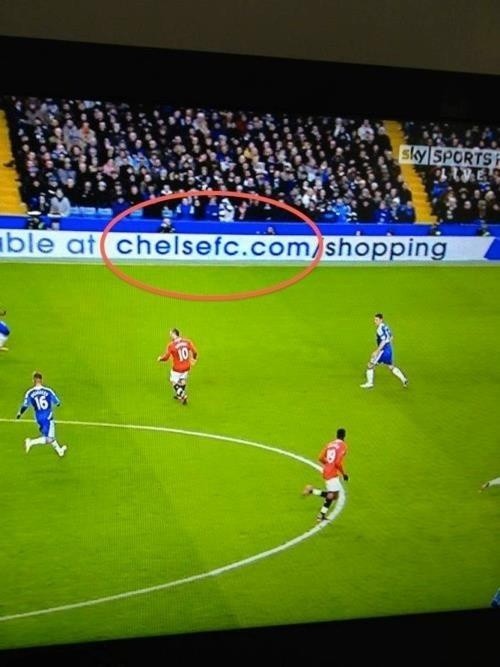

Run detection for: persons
[[16, 372, 67, 458], [0, 311, 9, 351], [302, 428, 349, 522], [359, 314, 408, 388], [481, 477, 500, 489], [157, 328, 197, 405]]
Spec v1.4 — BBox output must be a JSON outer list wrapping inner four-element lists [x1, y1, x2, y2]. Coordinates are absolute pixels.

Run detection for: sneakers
[[302, 484, 312, 495], [174, 395, 188, 404], [403, 379, 408, 388], [315, 514, 328, 521], [360, 383, 373, 388], [59, 445, 67, 457], [25, 438, 31, 453]]
